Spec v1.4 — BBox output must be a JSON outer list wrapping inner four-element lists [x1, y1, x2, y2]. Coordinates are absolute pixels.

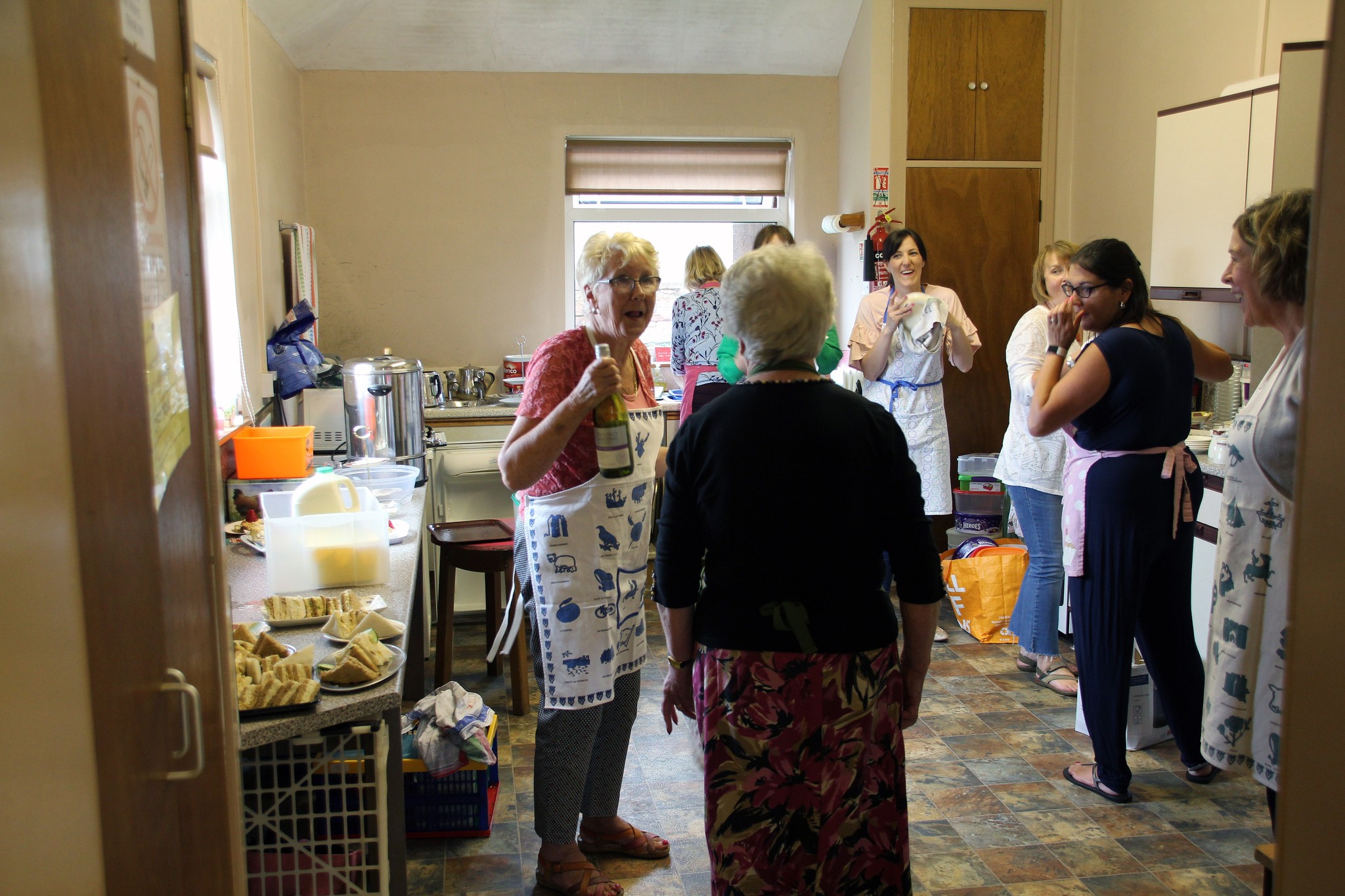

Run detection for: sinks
[[663, 392, 682, 401]]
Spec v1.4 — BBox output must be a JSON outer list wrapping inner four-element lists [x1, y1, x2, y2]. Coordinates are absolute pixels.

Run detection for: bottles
[[591, 343, 634, 479], [291, 466, 361, 517]]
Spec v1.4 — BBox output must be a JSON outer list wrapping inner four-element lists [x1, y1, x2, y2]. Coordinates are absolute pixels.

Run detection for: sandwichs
[[317, 628, 396, 683], [234, 623, 322, 710], [321, 609, 404, 640], [262, 589, 365, 619]]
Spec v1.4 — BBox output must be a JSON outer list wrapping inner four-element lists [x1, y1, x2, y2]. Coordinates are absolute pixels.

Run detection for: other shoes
[[933, 627, 947, 641]]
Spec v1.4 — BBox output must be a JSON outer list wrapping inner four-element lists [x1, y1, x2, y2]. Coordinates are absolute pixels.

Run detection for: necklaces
[[617, 352, 638, 401]]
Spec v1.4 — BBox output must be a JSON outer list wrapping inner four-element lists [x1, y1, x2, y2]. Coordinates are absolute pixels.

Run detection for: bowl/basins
[[899, 292, 929, 332], [953, 492, 1005, 515], [378, 499, 402, 519], [332, 465, 420, 508], [1191, 412, 1212, 423], [654, 386, 664, 398]]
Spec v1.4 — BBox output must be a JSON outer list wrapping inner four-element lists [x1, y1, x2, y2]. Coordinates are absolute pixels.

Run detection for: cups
[[1207, 421, 1233, 465], [324, 462, 342, 488]]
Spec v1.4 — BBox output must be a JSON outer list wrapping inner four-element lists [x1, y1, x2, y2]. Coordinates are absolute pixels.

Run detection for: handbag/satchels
[[939, 537, 1030, 644]]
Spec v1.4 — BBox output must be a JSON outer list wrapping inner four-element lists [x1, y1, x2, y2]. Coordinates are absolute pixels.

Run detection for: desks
[[217, 479, 429, 896]]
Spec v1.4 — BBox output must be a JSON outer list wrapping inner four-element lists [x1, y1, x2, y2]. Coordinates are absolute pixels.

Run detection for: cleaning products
[[652, 362, 667, 393]]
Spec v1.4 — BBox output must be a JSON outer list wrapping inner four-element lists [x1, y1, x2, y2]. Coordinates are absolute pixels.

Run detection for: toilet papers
[[822, 214, 850, 234]]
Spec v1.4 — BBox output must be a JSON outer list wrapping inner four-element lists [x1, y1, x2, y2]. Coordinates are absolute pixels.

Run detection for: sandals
[[1032, 664, 1078, 696], [1016, 651, 1078, 676], [577, 824, 669, 859], [535, 851, 625, 896]]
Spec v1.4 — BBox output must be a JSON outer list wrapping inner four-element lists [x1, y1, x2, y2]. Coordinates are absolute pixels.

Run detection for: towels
[[402, 678, 497, 778]]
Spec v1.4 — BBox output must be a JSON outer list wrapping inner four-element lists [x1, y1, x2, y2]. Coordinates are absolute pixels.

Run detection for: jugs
[[422, 371, 445, 407]]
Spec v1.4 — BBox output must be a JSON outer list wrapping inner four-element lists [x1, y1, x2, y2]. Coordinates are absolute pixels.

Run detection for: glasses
[[1061, 283, 1115, 298], [594, 276, 661, 294]]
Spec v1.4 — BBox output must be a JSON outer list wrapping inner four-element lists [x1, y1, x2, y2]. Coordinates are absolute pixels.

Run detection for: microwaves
[[303, 388, 347, 450]]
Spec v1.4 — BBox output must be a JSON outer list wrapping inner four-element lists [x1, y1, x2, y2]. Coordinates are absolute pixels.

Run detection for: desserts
[[235, 509, 264, 532], [248, 522, 265, 546]]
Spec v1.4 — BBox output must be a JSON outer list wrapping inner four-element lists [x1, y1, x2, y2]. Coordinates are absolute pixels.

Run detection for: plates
[[283, 643, 296, 655], [1184, 429, 1213, 454], [655, 395, 664, 400], [224, 519, 264, 534], [239, 692, 324, 717], [232, 621, 271, 636], [313, 643, 407, 692], [389, 519, 410, 544], [323, 619, 406, 642], [260, 595, 388, 626], [240, 535, 266, 557]]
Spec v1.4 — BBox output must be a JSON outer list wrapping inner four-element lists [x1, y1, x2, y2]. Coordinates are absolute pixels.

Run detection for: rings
[[1051, 318, 1057, 322], [676, 703, 683, 708]]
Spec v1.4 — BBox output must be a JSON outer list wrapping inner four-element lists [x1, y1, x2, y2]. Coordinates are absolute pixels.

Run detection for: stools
[[431, 519, 532, 717]]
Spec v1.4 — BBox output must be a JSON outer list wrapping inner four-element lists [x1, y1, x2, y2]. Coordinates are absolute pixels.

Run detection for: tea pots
[[442, 363, 495, 400]]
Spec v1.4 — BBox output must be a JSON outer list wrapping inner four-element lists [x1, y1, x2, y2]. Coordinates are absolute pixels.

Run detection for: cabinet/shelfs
[[421, 411, 681, 661], [906, 7, 1048, 161], [1148, 40, 1331, 304]]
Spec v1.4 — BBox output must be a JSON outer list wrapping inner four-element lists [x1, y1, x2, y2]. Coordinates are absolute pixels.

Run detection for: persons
[[993, 239, 1100, 695], [497, 231, 670, 896], [717, 225, 841, 385], [671, 245, 744, 433], [1198, 187, 1321, 872], [847, 227, 983, 643], [653, 238, 942, 896], [1028, 238, 1234, 802]]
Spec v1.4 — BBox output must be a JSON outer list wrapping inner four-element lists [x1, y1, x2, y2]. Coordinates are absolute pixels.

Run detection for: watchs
[[1065, 354, 1075, 369], [1044, 344, 1068, 357], [667, 651, 694, 669]]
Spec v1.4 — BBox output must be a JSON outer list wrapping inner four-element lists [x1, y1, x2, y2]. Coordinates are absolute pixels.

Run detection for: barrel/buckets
[[340, 354, 444, 488]]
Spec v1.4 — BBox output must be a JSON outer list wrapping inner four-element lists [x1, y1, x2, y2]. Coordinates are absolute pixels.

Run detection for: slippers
[[1186, 763, 1222, 784], [1063, 763, 1132, 803]]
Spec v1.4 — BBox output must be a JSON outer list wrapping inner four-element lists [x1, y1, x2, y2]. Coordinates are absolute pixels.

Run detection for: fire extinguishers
[[863, 207, 903, 293]]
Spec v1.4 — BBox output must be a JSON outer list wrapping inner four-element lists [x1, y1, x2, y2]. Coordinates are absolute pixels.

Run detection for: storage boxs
[[945, 452, 1006, 551], [231, 426, 317, 480], [1075, 637, 1174, 751], [308, 714, 500, 842]]
